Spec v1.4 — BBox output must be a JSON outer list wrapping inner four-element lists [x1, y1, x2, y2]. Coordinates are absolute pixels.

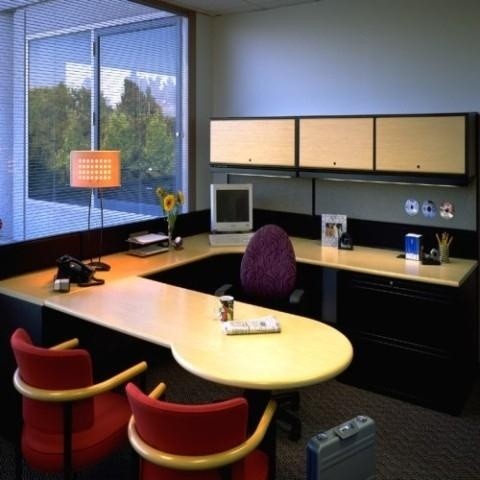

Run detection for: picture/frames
[[321, 214, 347, 246]]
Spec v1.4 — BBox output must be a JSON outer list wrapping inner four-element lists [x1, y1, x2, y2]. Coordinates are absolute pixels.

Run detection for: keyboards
[[207, 232, 256, 247]]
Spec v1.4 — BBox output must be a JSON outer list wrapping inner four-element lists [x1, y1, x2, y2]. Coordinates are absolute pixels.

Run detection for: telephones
[[55, 254, 94, 283]]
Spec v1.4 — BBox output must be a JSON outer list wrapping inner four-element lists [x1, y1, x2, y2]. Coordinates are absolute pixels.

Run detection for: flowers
[[156, 186, 185, 229]]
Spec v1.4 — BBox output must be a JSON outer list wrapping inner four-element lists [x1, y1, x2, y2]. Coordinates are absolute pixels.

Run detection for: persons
[[324, 222, 342, 248]]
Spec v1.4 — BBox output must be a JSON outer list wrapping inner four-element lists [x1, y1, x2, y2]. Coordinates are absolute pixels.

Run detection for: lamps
[[71, 151, 122, 270]]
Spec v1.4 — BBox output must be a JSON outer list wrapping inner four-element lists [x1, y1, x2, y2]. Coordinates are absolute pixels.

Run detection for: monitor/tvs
[[209, 183, 254, 232]]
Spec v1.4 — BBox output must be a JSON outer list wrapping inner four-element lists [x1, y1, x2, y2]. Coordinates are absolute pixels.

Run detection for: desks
[[0, 233, 478, 480]]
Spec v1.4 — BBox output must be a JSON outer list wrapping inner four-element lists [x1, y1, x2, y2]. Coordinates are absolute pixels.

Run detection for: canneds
[[219, 295, 234, 322]]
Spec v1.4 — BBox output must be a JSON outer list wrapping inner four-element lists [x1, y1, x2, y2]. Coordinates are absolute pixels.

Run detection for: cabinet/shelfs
[[209, 113, 480, 187]]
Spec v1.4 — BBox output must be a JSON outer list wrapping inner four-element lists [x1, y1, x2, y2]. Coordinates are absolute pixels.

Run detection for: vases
[[168, 216, 175, 243]]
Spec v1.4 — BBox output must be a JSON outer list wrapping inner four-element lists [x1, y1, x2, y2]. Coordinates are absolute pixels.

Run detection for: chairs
[[125, 382, 278, 480], [9, 328, 148, 480], [214, 224, 305, 443]]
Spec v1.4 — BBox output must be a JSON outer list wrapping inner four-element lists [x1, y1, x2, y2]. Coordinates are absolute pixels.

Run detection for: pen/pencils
[[435, 231, 454, 245]]
